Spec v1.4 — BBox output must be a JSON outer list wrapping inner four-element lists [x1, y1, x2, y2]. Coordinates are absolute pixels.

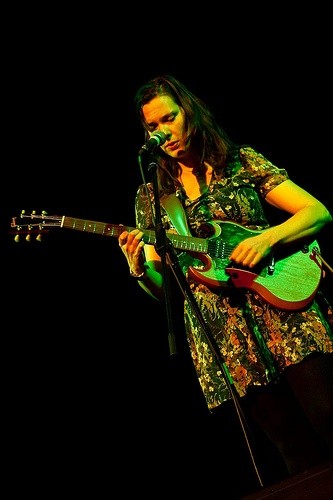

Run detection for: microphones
[[137, 129, 167, 158]]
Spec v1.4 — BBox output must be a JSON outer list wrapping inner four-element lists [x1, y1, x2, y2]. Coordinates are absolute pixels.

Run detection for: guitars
[[8, 209, 322, 312]]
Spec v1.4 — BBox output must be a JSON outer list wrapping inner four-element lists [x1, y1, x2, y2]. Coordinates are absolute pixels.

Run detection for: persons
[[118, 75, 333, 476]]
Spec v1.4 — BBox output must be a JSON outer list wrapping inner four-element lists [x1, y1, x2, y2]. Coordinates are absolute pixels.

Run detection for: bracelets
[[129, 264, 147, 281]]
